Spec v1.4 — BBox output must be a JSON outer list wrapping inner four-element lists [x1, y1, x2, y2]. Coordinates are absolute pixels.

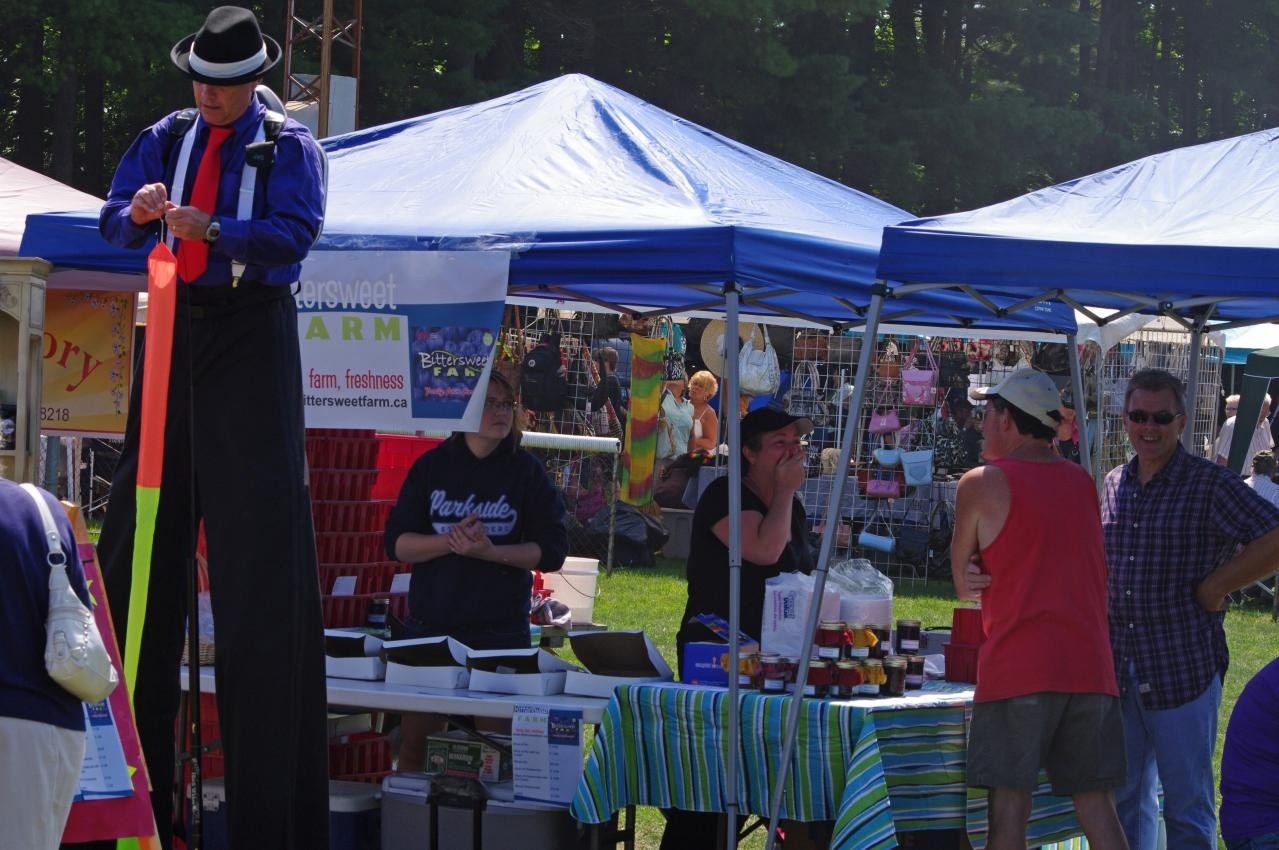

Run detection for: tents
[[296, 73, 915, 850], [1069, 307, 1226, 482], [1196, 323, 1279, 395], [0, 156, 162, 483], [774, 124, 1279, 850]]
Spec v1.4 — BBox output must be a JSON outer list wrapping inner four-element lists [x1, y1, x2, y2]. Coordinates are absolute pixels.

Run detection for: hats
[[741, 407, 814, 438], [170, 7, 282, 87], [969, 369, 1064, 430]]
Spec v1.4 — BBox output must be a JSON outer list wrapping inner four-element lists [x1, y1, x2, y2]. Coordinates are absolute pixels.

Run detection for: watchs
[[201, 214, 222, 245]]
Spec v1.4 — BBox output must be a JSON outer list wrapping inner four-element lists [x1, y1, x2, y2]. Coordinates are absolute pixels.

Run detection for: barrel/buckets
[[530, 556, 600, 626]]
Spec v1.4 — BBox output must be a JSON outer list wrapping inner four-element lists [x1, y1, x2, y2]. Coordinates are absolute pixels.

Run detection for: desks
[[178, 665, 978, 850]]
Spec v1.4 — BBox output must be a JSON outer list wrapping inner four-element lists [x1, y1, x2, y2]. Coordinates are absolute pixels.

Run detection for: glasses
[[484, 399, 517, 413], [1127, 409, 1183, 425]]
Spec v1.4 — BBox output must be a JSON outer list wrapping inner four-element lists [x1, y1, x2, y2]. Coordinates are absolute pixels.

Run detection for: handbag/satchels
[[23, 482, 116, 710], [738, 323, 780, 397], [856, 338, 971, 570], [811, 503, 851, 547], [784, 360, 830, 426]]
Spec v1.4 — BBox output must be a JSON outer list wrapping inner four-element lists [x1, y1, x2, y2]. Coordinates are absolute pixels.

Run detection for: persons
[[589, 346, 631, 435], [945, 369, 1131, 850], [101, 7, 331, 850], [1215, 392, 1279, 510], [1219, 657, 1279, 850], [675, 405, 814, 679], [1098, 369, 1279, 850], [1052, 386, 1113, 479], [0, 475, 93, 850], [650, 369, 719, 507], [379, 369, 569, 773]]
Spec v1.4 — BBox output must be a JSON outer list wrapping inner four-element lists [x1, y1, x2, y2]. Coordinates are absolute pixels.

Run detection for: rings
[[173, 225, 176, 231]]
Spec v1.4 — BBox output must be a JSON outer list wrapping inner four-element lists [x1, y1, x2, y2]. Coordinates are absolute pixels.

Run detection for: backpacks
[[520, 332, 570, 412]]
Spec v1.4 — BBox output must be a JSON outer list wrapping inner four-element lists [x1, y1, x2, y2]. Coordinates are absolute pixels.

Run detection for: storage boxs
[[678, 611, 760, 687], [424, 728, 512, 783], [385, 628, 669, 701], [319, 627, 384, 684], [940, 604, 986, 685]]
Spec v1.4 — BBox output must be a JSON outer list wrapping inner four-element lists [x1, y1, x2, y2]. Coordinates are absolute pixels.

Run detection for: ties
[[176, 126, 235, 283]]
